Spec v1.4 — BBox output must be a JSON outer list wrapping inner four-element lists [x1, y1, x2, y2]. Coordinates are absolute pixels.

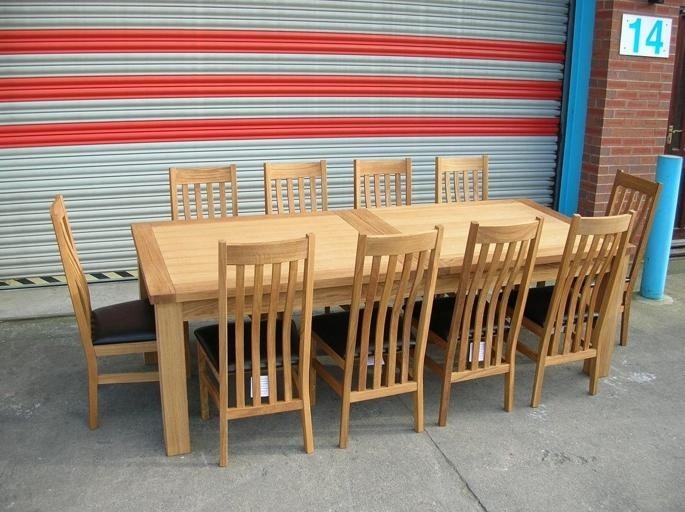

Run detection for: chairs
[[50, 193, 157, 429], [493, 209, 638, 408], [403, 215, 544, 427], [169, 163, 238, 379], [435, 155, 489, 203], [354, 158, 413, 305], [563, 169, 662, 347], [264, 159, 351, 314], [310, 223, 444, 449], [194, 233, 317, 467]]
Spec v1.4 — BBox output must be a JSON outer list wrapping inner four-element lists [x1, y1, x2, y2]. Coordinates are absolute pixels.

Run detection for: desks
[[130, 198, 637, 457]]
